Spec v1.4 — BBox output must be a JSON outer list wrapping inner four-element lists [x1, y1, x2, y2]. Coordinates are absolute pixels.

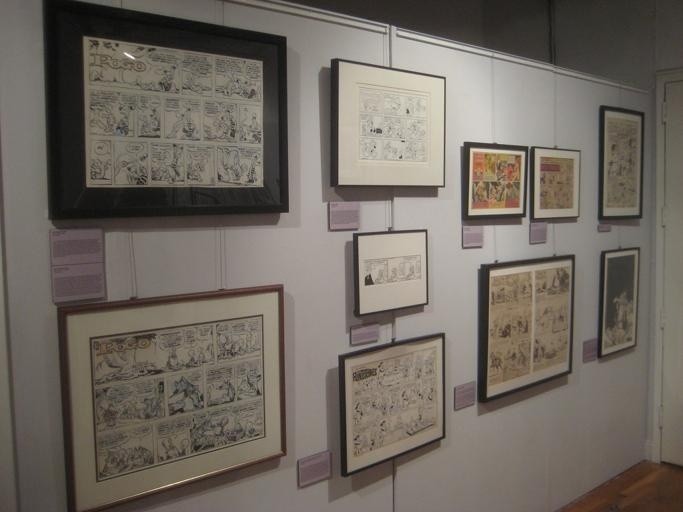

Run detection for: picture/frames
[[598, 247, 640, 357], [353, 229, 428, 318], [330, 58, 446, 187], [461, 142, 528, 219], [477, 254, 575, 403], [42, 0, 289, 220], [598, 105, 645, 220], [530, 146, 581, 219], [338, 333, 445, 477], [57, 284, 287, 512]]
